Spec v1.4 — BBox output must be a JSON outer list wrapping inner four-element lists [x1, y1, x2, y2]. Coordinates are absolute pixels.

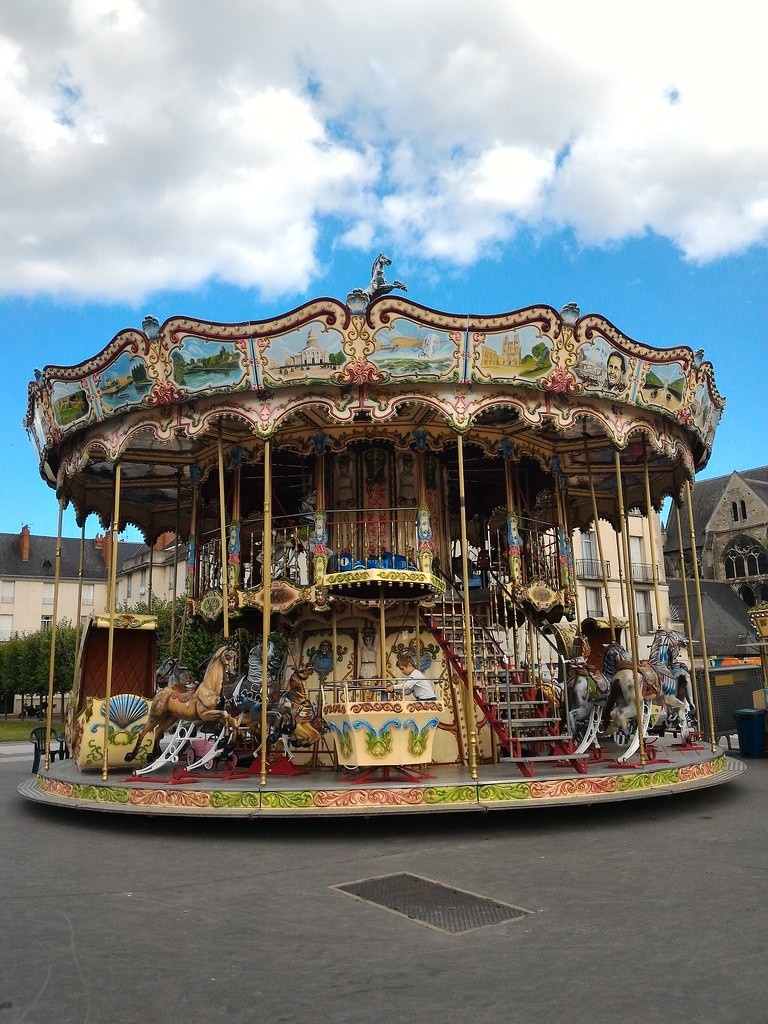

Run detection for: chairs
[[59, 732, 70, 759], [29, 727, 65, 774]]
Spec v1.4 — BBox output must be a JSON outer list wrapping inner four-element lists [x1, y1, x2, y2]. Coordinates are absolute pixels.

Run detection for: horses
[[525, 629, 696, 740], [123, 641, 321, 763], [253, 494, 332, 588]]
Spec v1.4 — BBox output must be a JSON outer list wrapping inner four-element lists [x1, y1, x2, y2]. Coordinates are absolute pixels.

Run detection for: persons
[[361, 634, 378, 678], [386, 655, 437, 701]]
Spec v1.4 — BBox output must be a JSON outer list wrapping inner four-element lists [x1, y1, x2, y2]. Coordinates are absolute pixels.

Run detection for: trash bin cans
[[732, 708, 766, 759]]
